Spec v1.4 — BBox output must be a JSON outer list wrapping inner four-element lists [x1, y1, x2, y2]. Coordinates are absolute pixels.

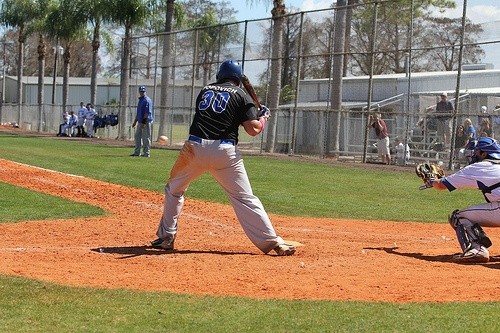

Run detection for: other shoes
[[129, 154, 150, 157], [150, 238, 174, 250], [453, 244, 490, 263], [274, 243, 296, 255]]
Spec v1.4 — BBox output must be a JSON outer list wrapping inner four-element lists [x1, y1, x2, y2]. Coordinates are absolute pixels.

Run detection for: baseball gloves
[[415, 163, 444, 190]]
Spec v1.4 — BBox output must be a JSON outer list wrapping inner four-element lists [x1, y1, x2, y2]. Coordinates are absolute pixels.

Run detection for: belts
[[189, 135, 234, 145]]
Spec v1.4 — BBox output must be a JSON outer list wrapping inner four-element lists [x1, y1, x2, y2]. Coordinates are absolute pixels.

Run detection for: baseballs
[[160, 136, 168, 144]]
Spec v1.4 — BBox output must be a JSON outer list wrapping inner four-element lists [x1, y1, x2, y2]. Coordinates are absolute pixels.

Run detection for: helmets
[[218, 59, 241, 80]]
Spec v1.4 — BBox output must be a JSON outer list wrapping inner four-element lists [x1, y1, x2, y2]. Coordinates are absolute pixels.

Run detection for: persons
[[455, 104, 500, 167], [61, 111, 78, 137], [129, 86, 154, 157], [368, 113, 391, 165], [77, 102, 88, 137], [151, 60, 306, 256], [416, 137, 500, 263], [392, 139, 410, 165], [57, 111, 71, 136], [436, 92, 455, 145], [85, 103, 97, 137]]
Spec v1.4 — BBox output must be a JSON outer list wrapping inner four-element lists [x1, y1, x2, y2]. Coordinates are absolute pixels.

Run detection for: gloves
[[257, 103, 271, 121]]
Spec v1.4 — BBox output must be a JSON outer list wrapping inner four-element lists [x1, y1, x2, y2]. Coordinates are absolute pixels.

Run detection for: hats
[[465, 137, 500, 160], [138, 86, 146, 92]]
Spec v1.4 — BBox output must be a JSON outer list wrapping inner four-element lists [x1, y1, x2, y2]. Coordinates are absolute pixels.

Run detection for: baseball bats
[[241, 73, 263, 112]]
